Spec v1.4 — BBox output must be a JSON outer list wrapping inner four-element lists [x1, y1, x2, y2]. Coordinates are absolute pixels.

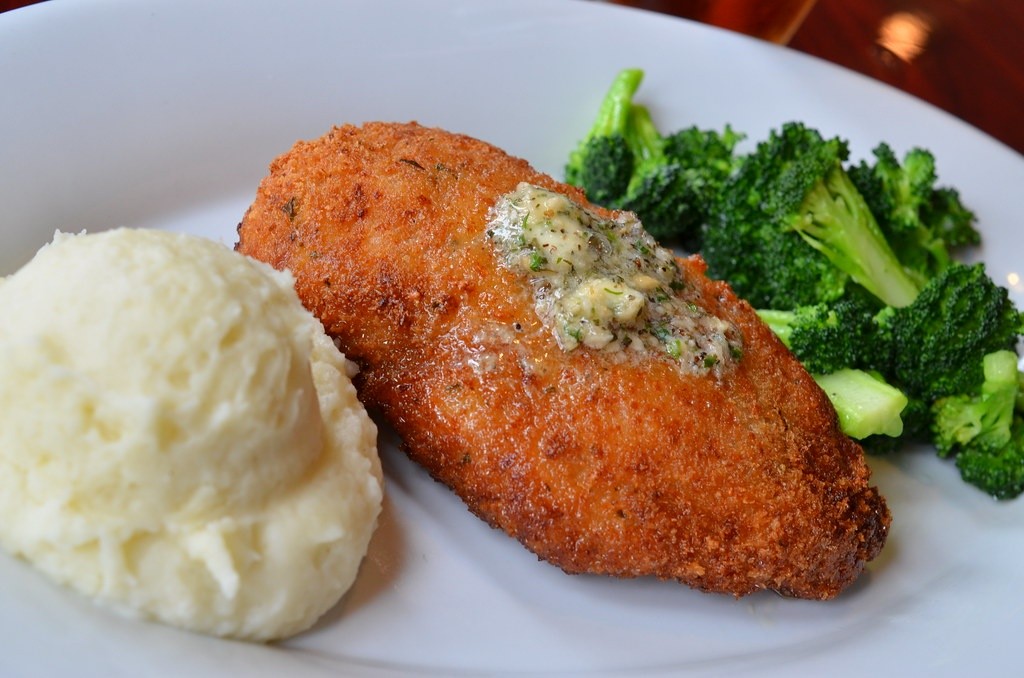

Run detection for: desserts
[[0, 225, 385, 643]]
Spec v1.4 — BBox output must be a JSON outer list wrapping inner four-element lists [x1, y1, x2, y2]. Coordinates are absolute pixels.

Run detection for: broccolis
[[562, 66, 1023, 503]]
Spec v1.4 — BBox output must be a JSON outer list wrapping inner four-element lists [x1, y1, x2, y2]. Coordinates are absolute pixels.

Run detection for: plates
[[0, 0, 1024, 678]]
